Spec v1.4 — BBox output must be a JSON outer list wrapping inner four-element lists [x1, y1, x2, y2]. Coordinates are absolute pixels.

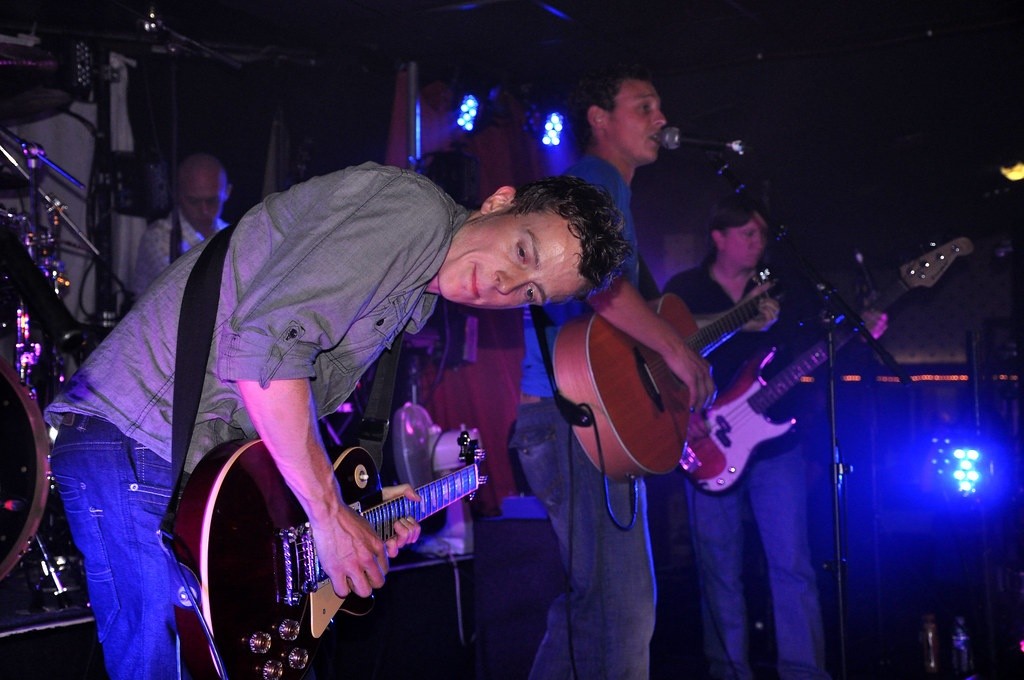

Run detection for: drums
[[0, 358, 59, 585]]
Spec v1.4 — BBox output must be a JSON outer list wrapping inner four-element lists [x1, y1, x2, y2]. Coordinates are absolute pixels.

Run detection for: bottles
[[951, 615, 974, 676], [923, 613, 940, 674]]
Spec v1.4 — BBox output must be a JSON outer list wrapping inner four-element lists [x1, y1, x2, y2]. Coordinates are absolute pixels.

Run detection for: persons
[[130, 62, 888, 680], [44, 158, 635, 680]]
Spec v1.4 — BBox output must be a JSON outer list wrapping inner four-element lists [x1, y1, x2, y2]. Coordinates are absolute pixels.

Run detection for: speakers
[[473, 495, 568, 680]]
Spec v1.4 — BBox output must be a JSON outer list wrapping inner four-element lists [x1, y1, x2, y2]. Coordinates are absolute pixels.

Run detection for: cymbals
[[0, 42, 84, 128]]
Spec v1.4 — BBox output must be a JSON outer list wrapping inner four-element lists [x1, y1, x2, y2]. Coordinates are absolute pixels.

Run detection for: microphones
[[661, 127, 748, 153]]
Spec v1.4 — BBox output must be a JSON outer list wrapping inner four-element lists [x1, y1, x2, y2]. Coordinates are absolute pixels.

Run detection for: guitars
[[550, 265, 786, 484], [682, 237, 977, 497], [169, 429, 493, 679]]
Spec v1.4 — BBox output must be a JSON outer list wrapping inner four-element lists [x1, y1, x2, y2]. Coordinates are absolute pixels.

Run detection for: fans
[[393, 401, 483, 554]]
